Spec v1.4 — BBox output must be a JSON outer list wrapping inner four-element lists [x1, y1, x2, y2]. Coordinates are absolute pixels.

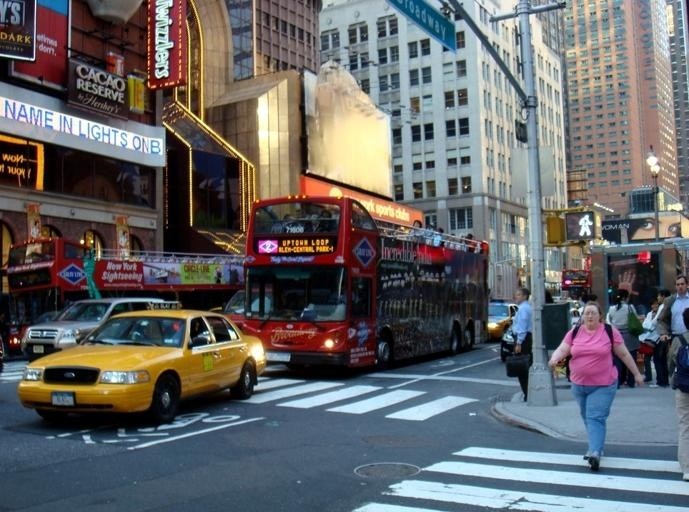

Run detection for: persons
[[316, 210, 337, 232], [511, 289, 533, 401], [407, 220, 476, 253], [330, 285, 356, 305], [548, 276, 689, 482]]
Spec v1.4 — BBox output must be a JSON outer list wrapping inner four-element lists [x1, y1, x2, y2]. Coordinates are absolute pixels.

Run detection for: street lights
[[647, 144, 661, 241]]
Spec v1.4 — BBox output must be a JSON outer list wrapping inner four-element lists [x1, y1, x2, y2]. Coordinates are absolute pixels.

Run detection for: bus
[[243, 195, 489, 370], [562, 269, 591, 297]]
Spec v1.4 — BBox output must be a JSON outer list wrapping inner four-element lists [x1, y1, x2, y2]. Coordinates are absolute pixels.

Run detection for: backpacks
[[671, 335, 689, 393]]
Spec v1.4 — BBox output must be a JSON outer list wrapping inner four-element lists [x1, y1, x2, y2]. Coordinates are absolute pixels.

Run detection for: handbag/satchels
[[628, 312, 643, 337], [505, 352, 529, 377]]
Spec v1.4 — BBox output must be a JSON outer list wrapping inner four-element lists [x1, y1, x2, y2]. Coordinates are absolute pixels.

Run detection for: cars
[[489, 301, 519, 337], [500, 324, 518, 361], [554, 296, 586, 326], [17, 308, 266, 423]]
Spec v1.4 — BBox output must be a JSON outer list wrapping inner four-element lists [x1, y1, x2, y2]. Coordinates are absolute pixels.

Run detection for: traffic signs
[[386, 0, 456, 53]]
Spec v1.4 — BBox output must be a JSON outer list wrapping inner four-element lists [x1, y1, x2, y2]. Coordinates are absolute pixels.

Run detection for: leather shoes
[[583, 454, 599, 472]]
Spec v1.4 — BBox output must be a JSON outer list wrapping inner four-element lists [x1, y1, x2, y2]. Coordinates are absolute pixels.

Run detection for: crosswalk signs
[[566, 211, 594, 239]]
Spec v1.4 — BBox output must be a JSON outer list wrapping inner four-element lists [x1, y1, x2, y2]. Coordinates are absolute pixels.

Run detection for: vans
[[221, 288, 272, 316]]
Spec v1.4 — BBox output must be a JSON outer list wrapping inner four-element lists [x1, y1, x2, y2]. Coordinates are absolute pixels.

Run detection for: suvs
[[20, 296, 181, 362]]
